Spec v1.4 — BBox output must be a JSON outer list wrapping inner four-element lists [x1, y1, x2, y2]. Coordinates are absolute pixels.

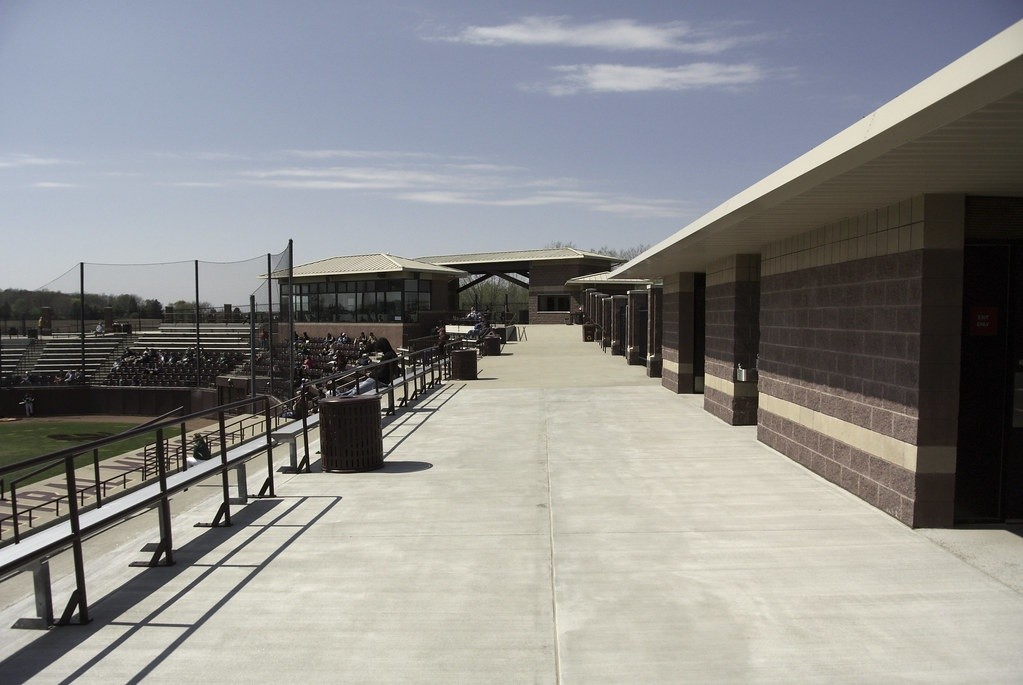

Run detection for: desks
[[514, 324, 535, 342]]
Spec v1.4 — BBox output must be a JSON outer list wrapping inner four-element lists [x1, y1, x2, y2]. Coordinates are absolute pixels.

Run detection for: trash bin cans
[[481, 334, 501, 357], [451, 348, 477, 380], [315, 395, 384, 473], [583, 324, 596, 343], [574, 311, 583, 325]]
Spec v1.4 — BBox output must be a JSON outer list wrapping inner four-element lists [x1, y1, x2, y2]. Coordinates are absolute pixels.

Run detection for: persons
[[470, 316, 489, 337], [186, 433, 211, 468], [95, 322, 105, 337], [38, 317, 44, 335], [423, 328, 449, 367], [4, 369, 83, 387], [342, 338, 399, 396], [244, 332, 377, 418], [23, 393, 35, 417], [106, 346, 238, 390]]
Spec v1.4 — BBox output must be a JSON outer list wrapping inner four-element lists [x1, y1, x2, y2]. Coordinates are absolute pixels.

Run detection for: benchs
[[0, 322, 493, 633]]
